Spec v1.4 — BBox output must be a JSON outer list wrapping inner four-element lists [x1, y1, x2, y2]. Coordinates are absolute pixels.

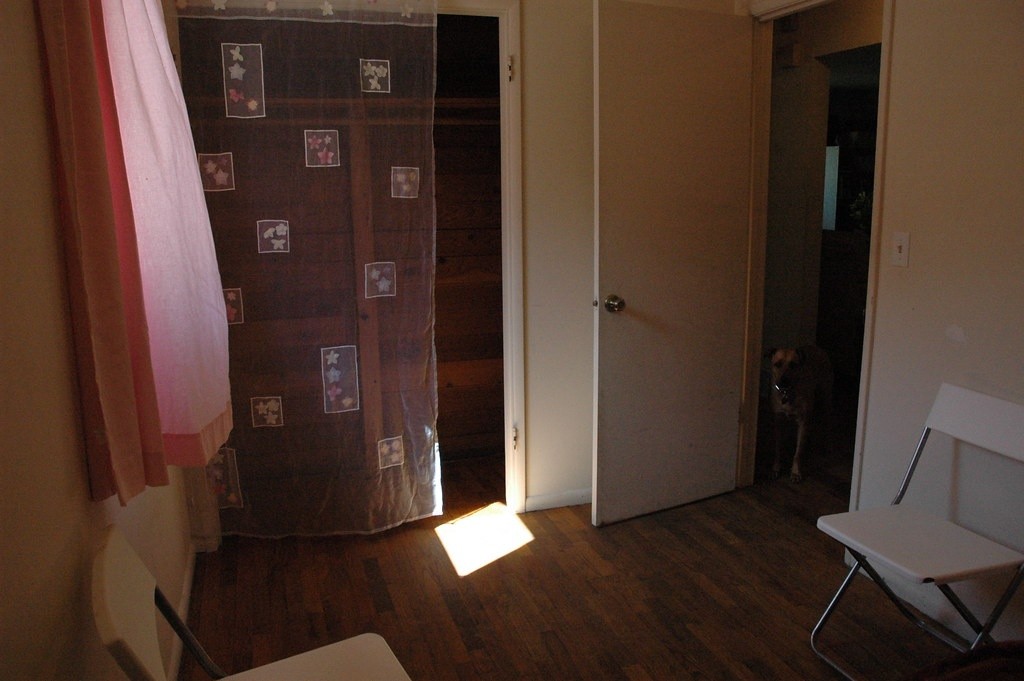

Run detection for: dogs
[[767, 345, 835, 484]]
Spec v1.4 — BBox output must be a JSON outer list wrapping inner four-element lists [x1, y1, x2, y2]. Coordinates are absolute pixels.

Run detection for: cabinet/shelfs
[[177, 15, 506, 517]]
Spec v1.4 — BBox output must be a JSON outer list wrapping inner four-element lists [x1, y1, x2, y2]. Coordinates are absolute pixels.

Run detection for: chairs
[[92, 523, 411, 681], [809, 383, 1024, 680]]
